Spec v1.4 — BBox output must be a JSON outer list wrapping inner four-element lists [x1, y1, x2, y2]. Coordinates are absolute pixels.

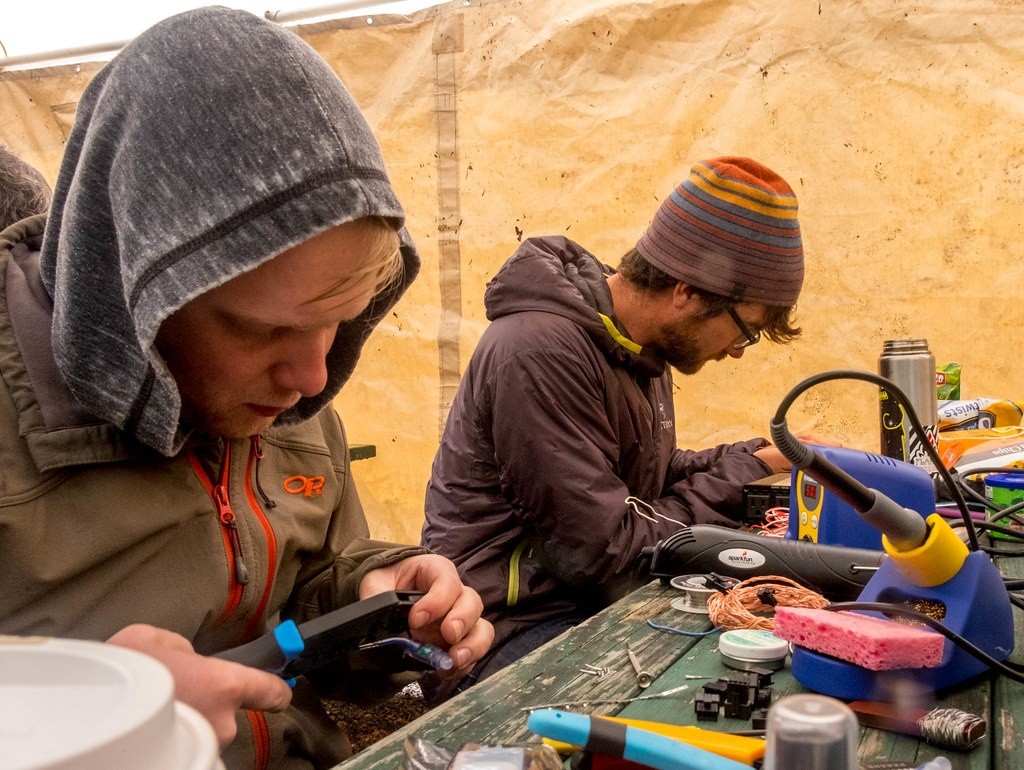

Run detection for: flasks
[[879, 339, 937, 476]]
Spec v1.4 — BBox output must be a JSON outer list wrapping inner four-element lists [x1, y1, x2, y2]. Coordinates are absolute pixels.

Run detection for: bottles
[[984, 474, 1024, 541]]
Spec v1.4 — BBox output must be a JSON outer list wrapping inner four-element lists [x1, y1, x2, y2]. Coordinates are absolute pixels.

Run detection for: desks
[[331, 518, 1024, 770]]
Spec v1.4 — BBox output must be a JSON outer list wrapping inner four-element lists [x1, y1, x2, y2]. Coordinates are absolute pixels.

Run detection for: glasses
[[721, 301, 761, 349]]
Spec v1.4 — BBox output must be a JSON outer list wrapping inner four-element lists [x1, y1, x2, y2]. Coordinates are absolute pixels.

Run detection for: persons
[[418, 156, 842, 710], [1, 4, 495, 770]]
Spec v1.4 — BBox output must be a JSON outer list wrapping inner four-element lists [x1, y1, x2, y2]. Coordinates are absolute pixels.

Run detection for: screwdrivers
[[624, 640, 652, 689]]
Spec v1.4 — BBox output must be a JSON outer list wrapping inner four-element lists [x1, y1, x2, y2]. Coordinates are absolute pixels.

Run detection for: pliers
[[205, 591, 427, 688]]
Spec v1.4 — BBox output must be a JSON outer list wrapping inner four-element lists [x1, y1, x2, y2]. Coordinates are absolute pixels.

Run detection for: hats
[[635, 156, 804, 307]]
